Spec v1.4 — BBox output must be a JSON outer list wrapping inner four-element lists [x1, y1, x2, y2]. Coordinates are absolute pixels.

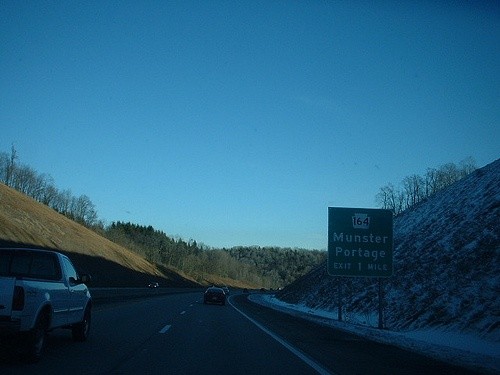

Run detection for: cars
[[204, 288, 226, 305], [148, 282, 160, 288], [222, 287, 230, 295]]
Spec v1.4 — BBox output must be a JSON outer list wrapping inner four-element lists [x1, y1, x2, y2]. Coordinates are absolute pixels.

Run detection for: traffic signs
[[328, 206, 394, 278]]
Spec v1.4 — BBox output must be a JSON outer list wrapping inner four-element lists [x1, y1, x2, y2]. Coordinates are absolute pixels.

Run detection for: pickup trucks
[[0, 248, 94, 361]]
